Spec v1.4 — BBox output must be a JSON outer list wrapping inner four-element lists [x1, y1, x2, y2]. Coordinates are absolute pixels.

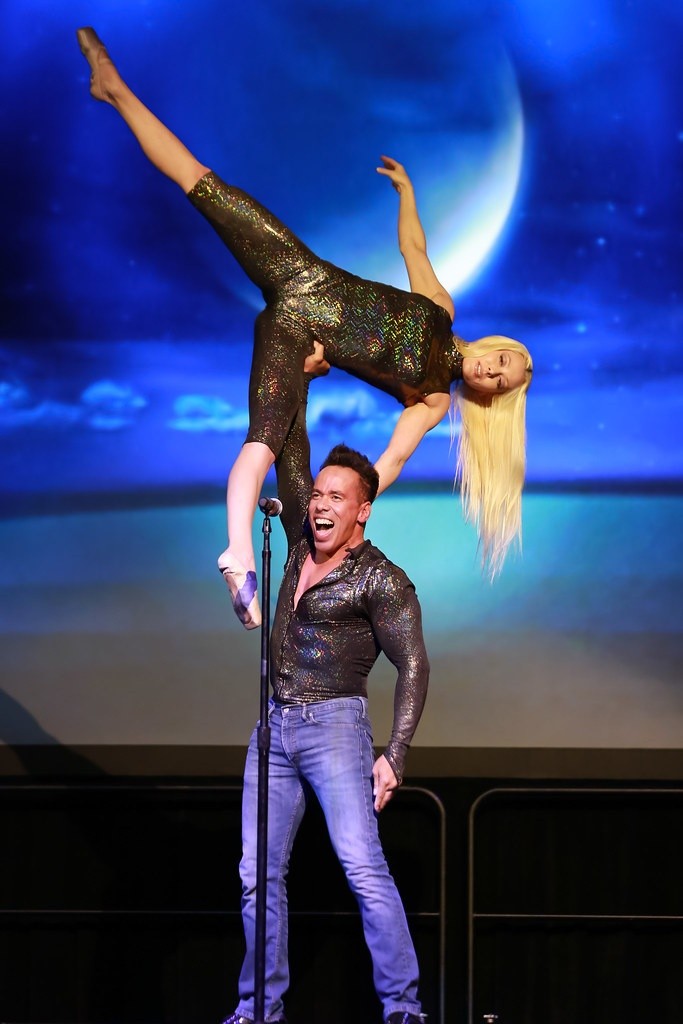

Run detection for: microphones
[[260, 495, 282, 518]]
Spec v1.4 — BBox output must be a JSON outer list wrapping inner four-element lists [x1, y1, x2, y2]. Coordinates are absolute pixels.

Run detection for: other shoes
[[78, 27, 110, 98], [218, 556, 263, 631]]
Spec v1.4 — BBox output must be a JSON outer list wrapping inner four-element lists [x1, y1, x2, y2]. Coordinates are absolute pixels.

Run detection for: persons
[[219, 330, 434, 1024], [77, 20, 538, 632]]
